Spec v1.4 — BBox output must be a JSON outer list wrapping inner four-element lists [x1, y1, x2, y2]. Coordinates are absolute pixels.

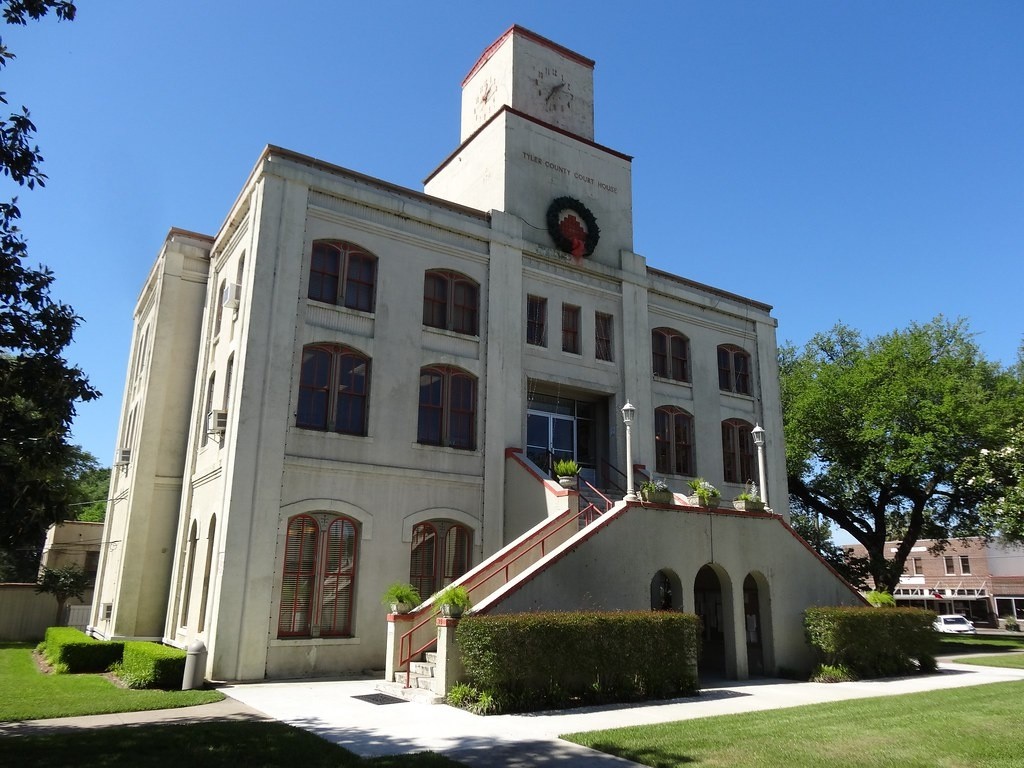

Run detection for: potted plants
[[553, 459, 582, 489], [686, 477, 722, 508], [639, 477, 673, 504], [380, 582, 422, 615], [430, 585, 473, 618], [865, 586, 895, 607], [731, 490, 766, 512]]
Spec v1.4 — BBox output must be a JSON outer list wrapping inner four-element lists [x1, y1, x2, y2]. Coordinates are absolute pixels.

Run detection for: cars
[[931, 612, 977, 637]]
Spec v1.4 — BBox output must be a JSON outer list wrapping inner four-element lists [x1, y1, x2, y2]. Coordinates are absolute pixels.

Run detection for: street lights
[[623, 396, 639, 502], [752, 418, 771, 513]]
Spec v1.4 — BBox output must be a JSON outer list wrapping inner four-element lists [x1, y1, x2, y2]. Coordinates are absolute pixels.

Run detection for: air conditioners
[[206, 410, 228, 433], [116, 448, 131, 466], [98, 603, 112, 620]]
